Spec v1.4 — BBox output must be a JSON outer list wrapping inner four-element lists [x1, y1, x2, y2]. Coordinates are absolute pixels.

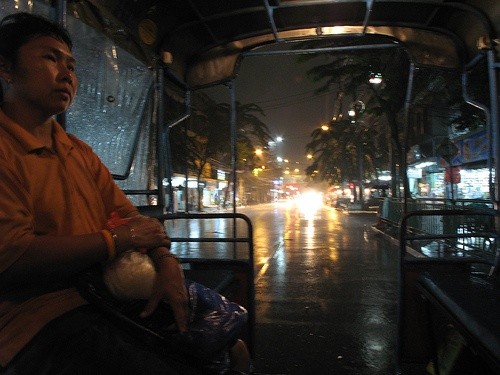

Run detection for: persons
[[0, 14, 255, 374]]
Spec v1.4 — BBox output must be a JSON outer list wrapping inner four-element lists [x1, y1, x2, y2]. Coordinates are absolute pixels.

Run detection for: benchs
[[396, 208, 500, 373], [157, 210, 262, 375]]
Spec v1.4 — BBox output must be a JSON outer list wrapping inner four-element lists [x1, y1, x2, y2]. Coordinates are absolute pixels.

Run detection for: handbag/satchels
[[72, 247, 246, 371]]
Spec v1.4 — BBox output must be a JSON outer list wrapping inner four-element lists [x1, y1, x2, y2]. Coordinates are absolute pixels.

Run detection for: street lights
[[251, 136, 320, 202], [347, 100, 368, 208], [321, 125, 346, 206]]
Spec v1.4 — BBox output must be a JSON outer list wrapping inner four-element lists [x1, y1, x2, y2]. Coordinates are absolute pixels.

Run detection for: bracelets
[[102, 228, 115, 261], [120, 220, 139, 251], [107, 226, 121, 260]]
